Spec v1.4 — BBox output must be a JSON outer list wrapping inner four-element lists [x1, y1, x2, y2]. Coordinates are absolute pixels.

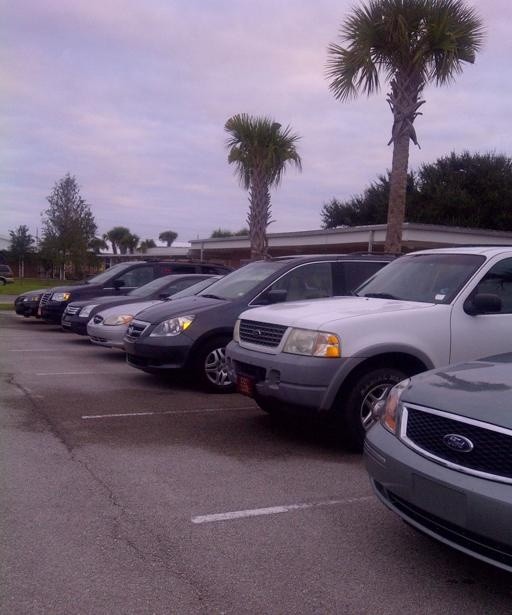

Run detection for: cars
[[365, 352, 512, 574], [86, 276, 226, 349], [0, 264, 14, 286], [14, 280, 82, 318], [61, 273, 227, 336], [37, 258, 235, 326]]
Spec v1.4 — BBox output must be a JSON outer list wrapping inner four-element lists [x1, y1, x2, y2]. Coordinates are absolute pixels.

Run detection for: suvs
[[222, 244, 511, 450], [122, 251, 408, 399]]
